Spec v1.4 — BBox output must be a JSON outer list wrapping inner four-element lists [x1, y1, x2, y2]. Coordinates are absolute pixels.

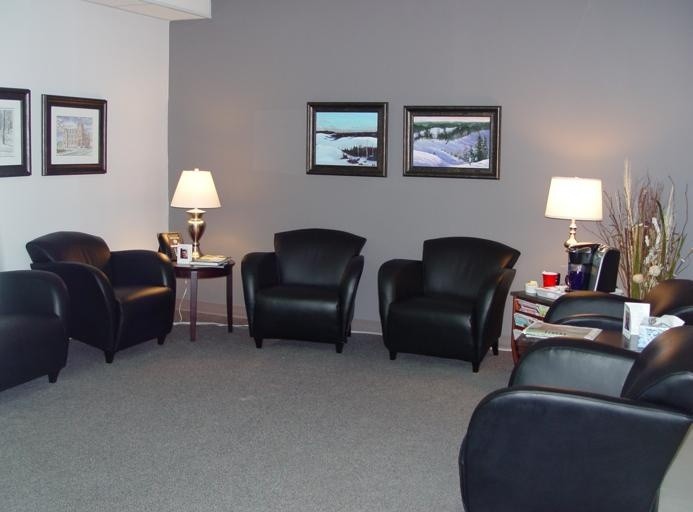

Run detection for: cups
[[542, 271, 559, 286], [524, 282, 539, 293]]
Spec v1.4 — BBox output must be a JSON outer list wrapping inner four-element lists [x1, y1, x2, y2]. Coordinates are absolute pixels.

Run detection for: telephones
[[157, 232, 184, 261]]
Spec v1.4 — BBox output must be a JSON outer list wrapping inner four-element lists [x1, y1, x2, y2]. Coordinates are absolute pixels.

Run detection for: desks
[[169, 254, 234, 342], [513, 323, 623, 365]]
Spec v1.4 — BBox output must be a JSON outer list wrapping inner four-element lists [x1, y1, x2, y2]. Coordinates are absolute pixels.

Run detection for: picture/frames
[[402, 105, 500, 180], [0, 87, 31, 177], [304, 101, 388, 178], [39, 95, 108, 176]]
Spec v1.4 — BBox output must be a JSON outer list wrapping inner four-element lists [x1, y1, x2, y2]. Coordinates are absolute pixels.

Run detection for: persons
[[180, 249, 187, 259]]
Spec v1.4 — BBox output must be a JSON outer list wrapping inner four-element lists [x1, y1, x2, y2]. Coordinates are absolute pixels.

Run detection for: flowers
[[592, 161, 691, 301]]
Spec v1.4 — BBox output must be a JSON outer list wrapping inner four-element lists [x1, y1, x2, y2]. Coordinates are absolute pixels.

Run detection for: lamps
[[544, 176, 604, 253], [169, 168, 220, 259]]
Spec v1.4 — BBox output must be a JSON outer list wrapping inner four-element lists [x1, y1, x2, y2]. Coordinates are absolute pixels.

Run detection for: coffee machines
[[566, 243, 620, 293]]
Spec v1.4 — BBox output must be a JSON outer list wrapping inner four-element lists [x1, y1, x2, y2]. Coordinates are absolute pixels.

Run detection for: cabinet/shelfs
[[508, 289, 552, 363]]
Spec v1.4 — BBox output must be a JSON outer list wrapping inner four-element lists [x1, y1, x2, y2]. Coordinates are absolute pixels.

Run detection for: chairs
[[25, 231, 177, 363], [455, 324, 692, 512], [376, 236, 521, 373], [239, 227, 367, 354], [0, 270, 71, 389], [540, 277, 692, 330]]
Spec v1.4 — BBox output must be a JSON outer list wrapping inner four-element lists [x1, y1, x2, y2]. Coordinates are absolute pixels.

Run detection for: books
[[509, 281, 568, 341], [522, 320, 602, 341], [190, 254, 231, 266], [191, 260, 229, 269]]
